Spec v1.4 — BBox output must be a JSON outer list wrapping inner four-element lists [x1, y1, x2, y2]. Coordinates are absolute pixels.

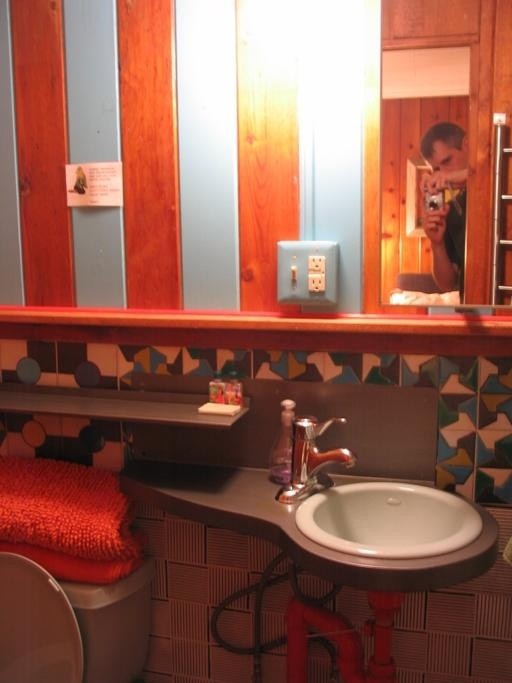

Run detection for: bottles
[[208, 371, 225, 405], [226, 371, 243, 405]]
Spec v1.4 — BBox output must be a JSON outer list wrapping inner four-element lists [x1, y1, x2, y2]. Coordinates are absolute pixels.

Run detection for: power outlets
[[277, 240, 338, 306]]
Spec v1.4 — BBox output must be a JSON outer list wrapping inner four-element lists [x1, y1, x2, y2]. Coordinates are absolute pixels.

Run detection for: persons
[[417, 120, 469, 297]]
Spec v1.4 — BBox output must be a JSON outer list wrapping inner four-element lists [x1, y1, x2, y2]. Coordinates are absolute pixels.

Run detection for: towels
[[1, 540, 141, 586], [0, 455, 149, 561]]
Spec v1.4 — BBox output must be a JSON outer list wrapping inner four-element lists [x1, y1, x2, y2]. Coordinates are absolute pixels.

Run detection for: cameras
[[424, 189, 445, 215]]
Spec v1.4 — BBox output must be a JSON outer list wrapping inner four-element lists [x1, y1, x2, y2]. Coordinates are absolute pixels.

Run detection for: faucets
[[291, 410, 355, 484]]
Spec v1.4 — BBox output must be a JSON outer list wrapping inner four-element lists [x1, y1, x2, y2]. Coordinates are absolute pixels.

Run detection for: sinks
[[294, 480, 479, 562]]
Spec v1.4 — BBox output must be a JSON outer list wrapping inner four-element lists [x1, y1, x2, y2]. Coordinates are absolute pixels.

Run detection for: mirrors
[[380, 0, 512, 308]]
